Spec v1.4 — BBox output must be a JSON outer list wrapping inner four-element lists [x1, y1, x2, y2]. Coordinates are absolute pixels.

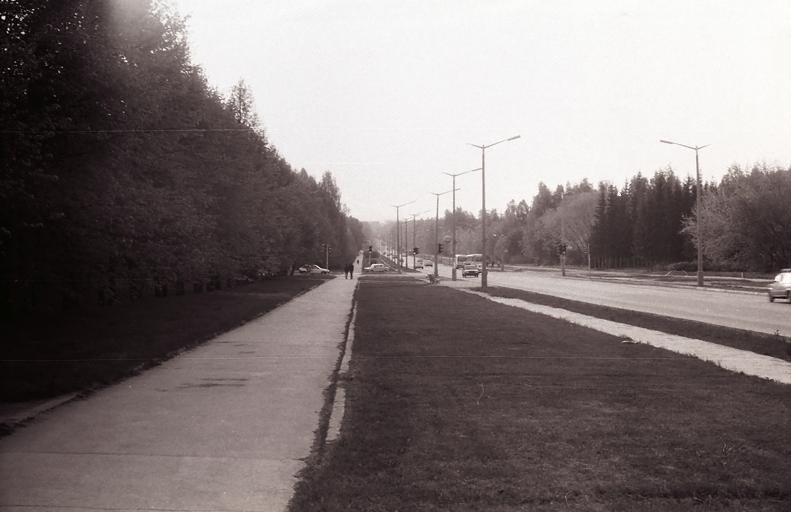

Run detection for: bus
[[455, 253, 484, 270]]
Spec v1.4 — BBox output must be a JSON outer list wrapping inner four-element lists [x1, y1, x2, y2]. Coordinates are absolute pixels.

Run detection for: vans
[[369, 264, 386, 272]]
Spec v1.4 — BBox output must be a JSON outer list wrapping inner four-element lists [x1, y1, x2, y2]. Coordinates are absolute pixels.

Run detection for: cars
[[766, 272, 791, 304], [298, 264, 330, 276], [461, 265, 479, 276]]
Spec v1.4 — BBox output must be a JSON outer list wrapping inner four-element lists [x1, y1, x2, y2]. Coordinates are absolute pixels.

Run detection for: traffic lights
[[439, 242, 444, 253], [558, 244, 566, 255]]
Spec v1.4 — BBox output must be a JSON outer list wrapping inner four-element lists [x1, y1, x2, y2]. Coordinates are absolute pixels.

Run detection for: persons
[[343, 263, 349, 279], [348, 263, 354, 280]]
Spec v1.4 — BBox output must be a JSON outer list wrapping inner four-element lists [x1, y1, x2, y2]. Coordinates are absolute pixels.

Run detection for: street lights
[[430, 187, 461, 277], [441, 166, 481, 281], [658, 139, 714, 288], [465, 133, 522, 290], [389, 196, 431, 273]]
